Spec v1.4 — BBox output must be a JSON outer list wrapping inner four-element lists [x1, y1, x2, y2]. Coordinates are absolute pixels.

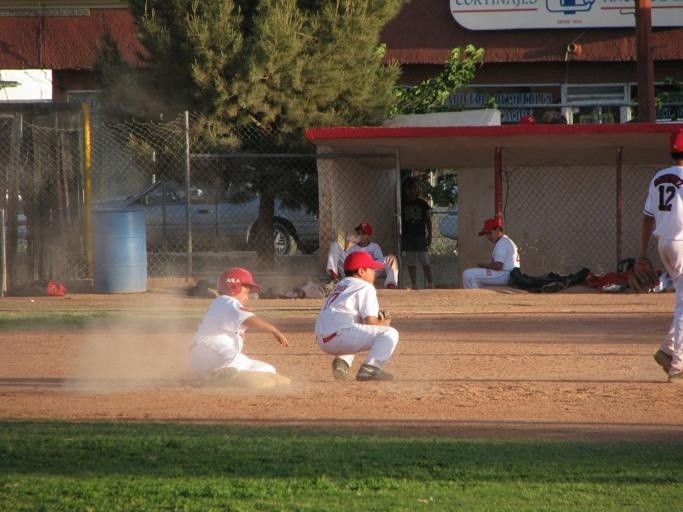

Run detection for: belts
[[322, 333, 338, 342]]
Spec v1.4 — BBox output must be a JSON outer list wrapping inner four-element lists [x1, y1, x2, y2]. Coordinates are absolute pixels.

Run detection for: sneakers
[[386, 284, 407, 290], [203, 369, 237, 388], [356, 365, 393, 381], [324, 279, 339, 293], [654, 351, 673, 374], [332, 359, 349, 379], [669, 371, 683, 383], [172, 369, 192, 387]]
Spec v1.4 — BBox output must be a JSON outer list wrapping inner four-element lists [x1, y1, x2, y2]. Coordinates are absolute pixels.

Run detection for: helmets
[[217, 268, 260, 295]]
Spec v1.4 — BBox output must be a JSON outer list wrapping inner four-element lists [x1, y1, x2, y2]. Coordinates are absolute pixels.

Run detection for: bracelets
[[637, 256, 647, 260]]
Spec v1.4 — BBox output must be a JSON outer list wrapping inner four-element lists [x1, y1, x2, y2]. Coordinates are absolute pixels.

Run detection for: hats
[[668, 129, 683, 151], [478, 219, 502, 236], [343, 251, 384, 271], [354, 223, 372, 234]]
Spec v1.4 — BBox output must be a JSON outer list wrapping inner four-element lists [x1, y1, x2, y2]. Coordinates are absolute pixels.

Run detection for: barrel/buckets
[[89, 206, 148, 293]]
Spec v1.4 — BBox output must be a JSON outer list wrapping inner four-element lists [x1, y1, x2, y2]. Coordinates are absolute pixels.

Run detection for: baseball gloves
[[626, 257, 661, 292], [379, 309, 390, 320]]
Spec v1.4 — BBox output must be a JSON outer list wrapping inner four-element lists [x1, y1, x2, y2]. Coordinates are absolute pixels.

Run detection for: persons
[[635, 126, 682, 385], [398, 174, 441, 289], [313, 251, 401, 382], [179, 265, 289, 385], [461, 217, 521, 291], [322, 222, 398, 290]]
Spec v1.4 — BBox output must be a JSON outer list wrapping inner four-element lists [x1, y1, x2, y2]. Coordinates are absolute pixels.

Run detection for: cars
[[87, 163, 319, 256]]
